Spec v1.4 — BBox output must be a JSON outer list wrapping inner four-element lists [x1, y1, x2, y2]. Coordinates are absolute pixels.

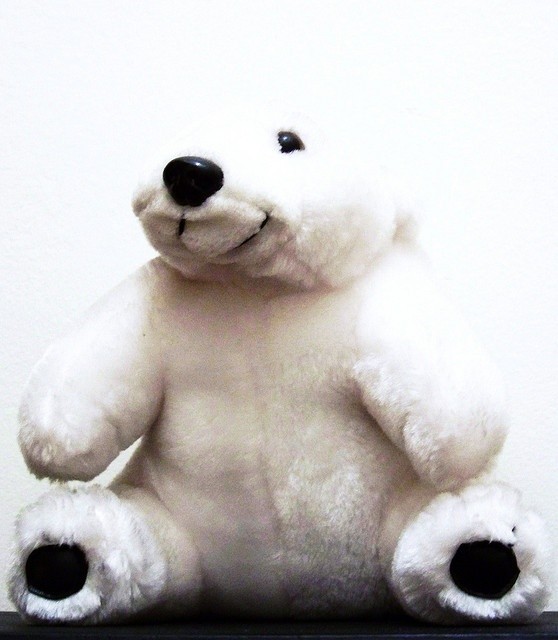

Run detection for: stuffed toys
[[6, 104, 553, 628]]
